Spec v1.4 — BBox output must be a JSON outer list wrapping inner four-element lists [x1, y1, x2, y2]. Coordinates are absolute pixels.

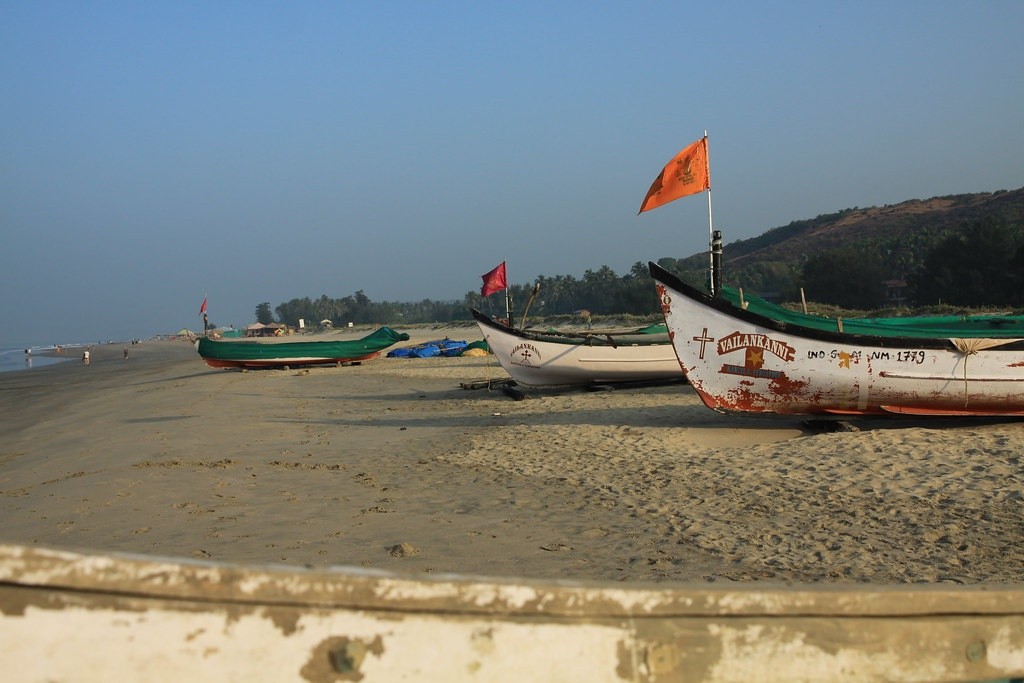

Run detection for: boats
[[470, 282, 830, 391], [188, 312, 411, 373], [648, 229, 1024, 420]]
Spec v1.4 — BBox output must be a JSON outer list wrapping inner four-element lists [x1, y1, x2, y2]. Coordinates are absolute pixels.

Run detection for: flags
[[637, 136, 710, 215], [480, 261, 508, 297], [199, 297, 207, 316]]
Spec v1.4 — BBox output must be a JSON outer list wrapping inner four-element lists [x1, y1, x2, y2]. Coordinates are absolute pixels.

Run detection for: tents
[[245, 323, 288, 337], [320, 319, 333, 328], [177, 328, 195, 338]]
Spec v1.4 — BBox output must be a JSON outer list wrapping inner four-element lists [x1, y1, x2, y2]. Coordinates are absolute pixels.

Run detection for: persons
[[132, 337, 141, 346], [24, 347, 32, 355], [82, 348, 90, 366], [123, 345, 128, 361], [56, 345, 63, 353]]
